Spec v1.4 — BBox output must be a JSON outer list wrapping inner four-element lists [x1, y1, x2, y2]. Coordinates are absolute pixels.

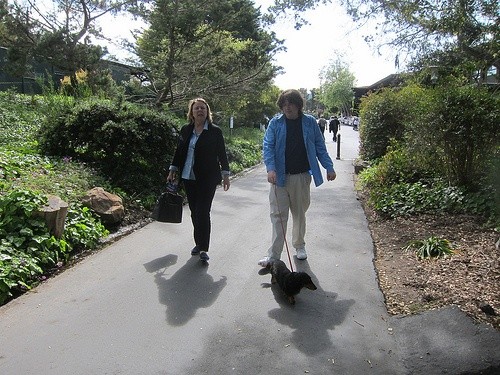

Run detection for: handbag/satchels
[[151, 181, 183, 223]]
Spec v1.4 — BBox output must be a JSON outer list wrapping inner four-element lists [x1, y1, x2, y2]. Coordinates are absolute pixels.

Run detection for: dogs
[[265, 258, 317, 304]]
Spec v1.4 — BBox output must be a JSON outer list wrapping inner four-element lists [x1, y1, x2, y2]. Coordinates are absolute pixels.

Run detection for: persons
[[169, 99, 230, 260], [258, 89, 336, 266], [318, 115, 340, 141]]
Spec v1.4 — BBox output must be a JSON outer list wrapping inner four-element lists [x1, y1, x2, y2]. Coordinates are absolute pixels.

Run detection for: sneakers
[[257, 256, 279, 266], [296, 248, 307, 260]]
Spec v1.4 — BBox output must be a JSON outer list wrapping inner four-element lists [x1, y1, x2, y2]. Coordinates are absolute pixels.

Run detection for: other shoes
[[191, 245, 202, 255], [200, 252, 209, 260]]
